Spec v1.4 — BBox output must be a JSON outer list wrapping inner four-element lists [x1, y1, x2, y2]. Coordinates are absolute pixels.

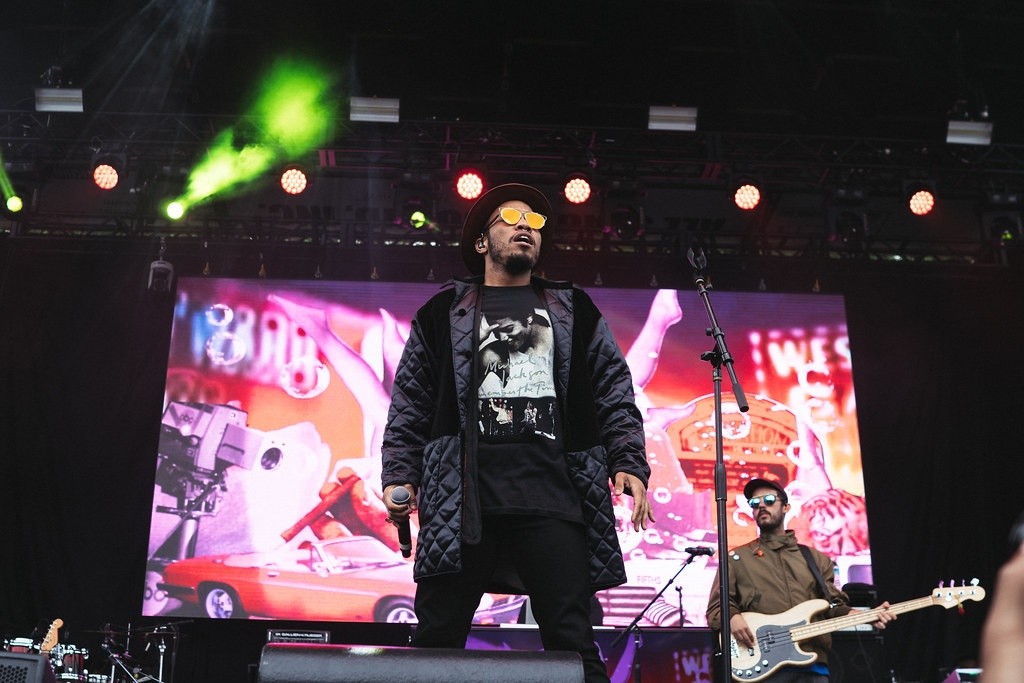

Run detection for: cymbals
[[87, 629, 136, 639], [142, 632, 185, 639]]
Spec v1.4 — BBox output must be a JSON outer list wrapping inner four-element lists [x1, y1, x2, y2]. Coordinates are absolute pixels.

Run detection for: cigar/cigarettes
[[281, 474, 361, 544]]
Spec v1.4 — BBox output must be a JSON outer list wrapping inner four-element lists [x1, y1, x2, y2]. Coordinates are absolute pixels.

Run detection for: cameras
[[154, 400, 288, 502]]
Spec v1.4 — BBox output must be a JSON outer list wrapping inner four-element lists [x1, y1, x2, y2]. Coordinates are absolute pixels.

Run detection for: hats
[[742, 478, 787, 502], [460, 182, 552, 274]]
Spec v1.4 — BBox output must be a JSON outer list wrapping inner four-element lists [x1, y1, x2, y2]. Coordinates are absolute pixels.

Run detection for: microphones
[[684, 547, 716, 556], [388, 485, 413, 559]]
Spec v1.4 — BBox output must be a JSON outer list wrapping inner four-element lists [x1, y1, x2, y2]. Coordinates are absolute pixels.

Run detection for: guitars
[[37, 618, 64, 660], [718, 577, 989, 682]]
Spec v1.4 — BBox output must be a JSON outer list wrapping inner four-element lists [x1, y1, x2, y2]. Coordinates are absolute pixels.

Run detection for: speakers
[[0, 653, 58, 683], [256, 643, 584, 683]]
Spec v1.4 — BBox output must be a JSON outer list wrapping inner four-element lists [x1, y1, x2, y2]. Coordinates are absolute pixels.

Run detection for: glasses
[[481, 207, 548, 229], [748, 494, 779, 509]]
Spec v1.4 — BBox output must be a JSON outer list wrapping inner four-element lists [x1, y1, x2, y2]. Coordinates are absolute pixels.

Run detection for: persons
[[382, 180, 657, 683], [980, 544, 1024, 682], [706, 477, 898, 683], [309, 466, 423, 556]]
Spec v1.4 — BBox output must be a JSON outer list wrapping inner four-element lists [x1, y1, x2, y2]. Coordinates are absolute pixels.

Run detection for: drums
[[86, 674, 120, 683], [5, 638, 36, 654], [51, 646, 89, 680]]
